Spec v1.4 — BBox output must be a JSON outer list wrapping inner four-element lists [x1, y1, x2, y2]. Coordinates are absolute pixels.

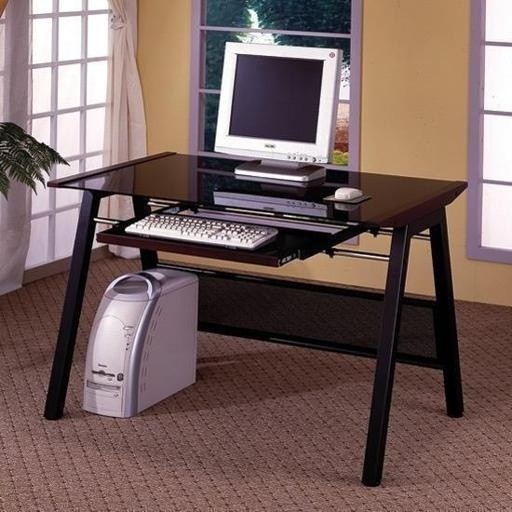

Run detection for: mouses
[[335, 189, 362, 201]]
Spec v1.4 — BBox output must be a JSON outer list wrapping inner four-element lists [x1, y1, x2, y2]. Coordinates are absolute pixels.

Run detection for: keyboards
[[123, 213, 277, 251]]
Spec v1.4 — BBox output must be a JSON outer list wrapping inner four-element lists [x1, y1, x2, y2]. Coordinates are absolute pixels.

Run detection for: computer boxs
[[82, 268, 200, 417]]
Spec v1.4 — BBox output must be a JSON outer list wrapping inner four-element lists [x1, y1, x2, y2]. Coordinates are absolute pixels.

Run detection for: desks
[[44, 151, 469, 487]]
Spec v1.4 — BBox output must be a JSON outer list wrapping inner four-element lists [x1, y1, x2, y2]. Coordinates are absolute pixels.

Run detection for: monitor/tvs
[[213, 41, 343, 183]]
